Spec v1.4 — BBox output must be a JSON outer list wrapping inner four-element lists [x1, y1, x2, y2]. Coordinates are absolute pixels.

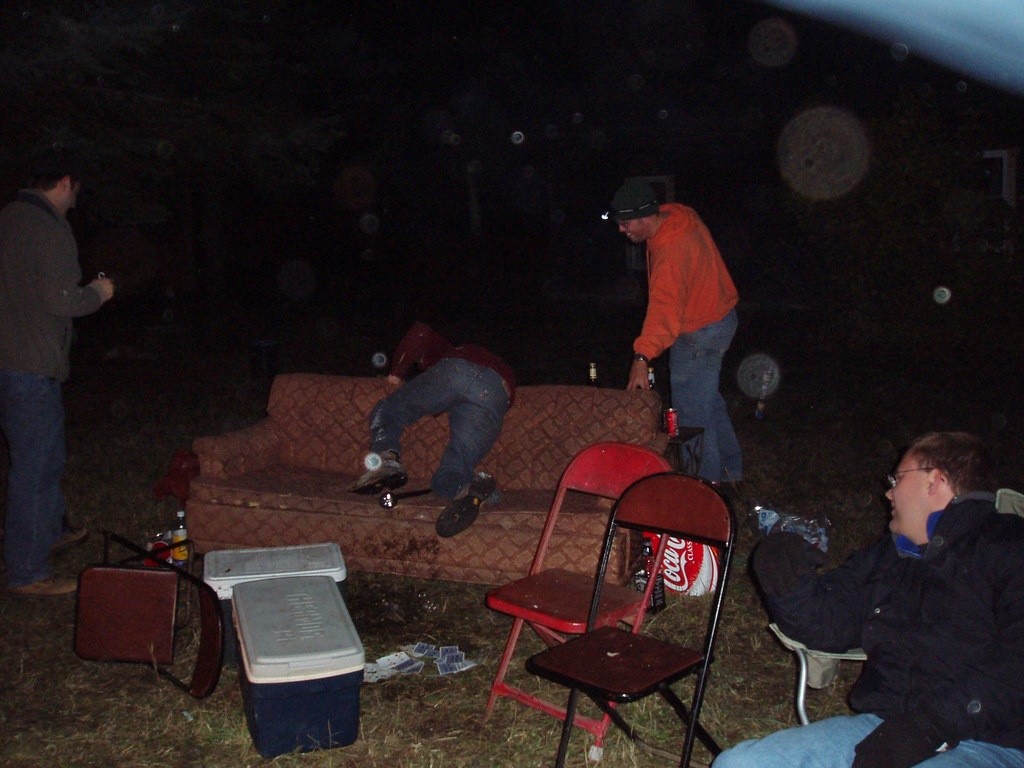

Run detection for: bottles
[[648, 367, 657, 391], [172, 511, 188, 562], [588, 363, 598, 385], [641, 538, 667, 611]]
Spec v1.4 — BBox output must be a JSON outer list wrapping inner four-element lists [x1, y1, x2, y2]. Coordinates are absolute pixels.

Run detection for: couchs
[[186, 372, 706, 588]]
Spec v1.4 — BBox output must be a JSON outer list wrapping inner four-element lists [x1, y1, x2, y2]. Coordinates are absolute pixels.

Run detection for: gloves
[[852, 701, 959, 768], [753, 531, 830, 597]]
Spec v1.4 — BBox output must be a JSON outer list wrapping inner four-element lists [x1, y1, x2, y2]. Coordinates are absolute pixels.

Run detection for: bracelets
[[633, 354, 648, 362]]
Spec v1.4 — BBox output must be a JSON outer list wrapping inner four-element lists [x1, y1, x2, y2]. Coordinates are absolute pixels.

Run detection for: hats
[[610, 184, 658, 219]]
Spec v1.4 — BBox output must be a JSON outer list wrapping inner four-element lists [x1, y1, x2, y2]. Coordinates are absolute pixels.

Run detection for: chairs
[[72, 530, 226, 699], [524, 471, 736, 768], [482, 442, 675, 764]]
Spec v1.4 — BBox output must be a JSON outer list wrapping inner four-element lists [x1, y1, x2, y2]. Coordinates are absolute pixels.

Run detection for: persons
[[343, 323, 517, 540], [609, 180, 757, 517], [707, 426, 1024, 767], [0, 155, 116, 599]]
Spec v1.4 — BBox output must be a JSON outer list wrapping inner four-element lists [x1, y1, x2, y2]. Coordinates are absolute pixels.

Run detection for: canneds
[[666, 408, 680, 437]]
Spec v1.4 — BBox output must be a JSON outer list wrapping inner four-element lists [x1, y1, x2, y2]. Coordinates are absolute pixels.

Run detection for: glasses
[[886, 468, 944, 488]]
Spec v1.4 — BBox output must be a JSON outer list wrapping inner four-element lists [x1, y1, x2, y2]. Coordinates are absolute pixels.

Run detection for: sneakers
[[45, 526, 90, 560], [436, 472, 496, 537], [346, 460, 408, 495], [7, 573, 79, 600]]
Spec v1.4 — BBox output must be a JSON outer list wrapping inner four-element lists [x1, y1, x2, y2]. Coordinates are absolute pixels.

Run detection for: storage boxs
[[641, 527, 721, 597], [232, 575, 366, 758], [204, 543, 346, 667]]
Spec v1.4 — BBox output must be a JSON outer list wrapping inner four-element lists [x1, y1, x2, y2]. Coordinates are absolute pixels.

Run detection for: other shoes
[[721, 481, 745, 497]]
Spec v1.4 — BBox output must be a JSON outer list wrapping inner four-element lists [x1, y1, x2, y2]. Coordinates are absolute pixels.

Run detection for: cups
[[143, 541, 172, 569]]
